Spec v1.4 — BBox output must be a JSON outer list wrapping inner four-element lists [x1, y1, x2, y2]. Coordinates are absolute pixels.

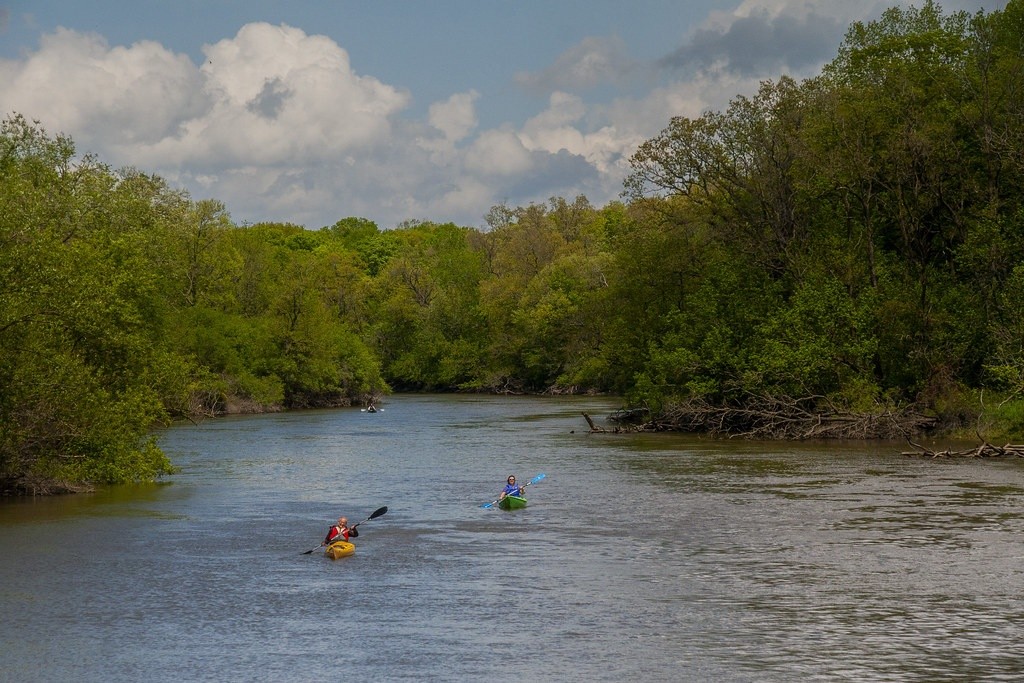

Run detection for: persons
[[367, 403, 375, 410], [320, 516, 358, 545], [499, 474, 524, 499]]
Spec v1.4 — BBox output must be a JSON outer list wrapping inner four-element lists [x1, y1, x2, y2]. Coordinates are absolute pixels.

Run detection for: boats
[[368, 410, 377, 413], [499, 494, 528, 511], [325, 541, 357, 560]]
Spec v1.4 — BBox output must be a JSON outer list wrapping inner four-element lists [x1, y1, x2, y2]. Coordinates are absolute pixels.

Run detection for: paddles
[[477, 473, 546, 509], [361, 408, 386, 411], [299, 505, 388, 557]]
